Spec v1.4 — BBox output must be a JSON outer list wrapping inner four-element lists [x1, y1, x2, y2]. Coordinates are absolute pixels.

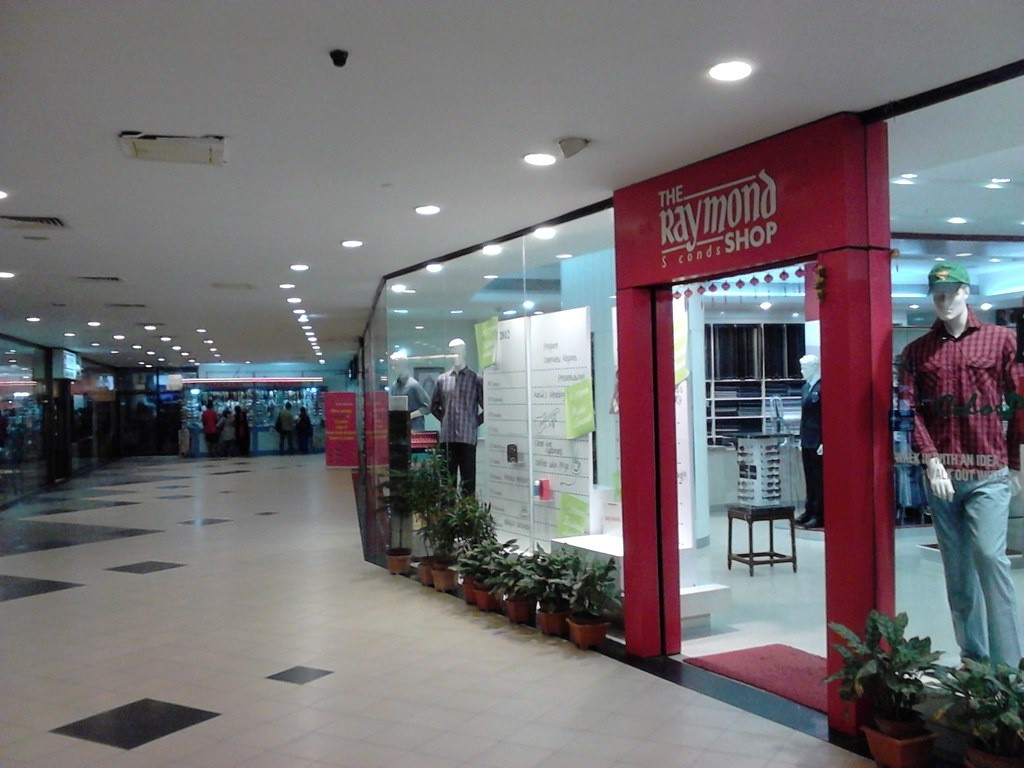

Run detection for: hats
[[927, 260, 970, 296]]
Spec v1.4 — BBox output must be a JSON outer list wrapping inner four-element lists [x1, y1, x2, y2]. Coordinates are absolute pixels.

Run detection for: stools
[[726, 505, 797, 577]]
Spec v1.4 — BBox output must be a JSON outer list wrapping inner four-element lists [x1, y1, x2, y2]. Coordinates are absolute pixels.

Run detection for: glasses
[[737, 446, 756, 501], [764, 444, 782, 500]]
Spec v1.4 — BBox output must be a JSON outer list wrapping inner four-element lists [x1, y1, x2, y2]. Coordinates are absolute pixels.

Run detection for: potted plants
[[819, 610, 939, 768], [376, 439, 625, 650], [926, 649, 1024, 768]]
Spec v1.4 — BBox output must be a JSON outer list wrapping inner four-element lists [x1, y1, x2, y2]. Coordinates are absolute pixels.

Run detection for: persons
[[295, 406, 312, 455], [230, 406, 251, 457], [216, 408, 236, 457], [277, 402, 295, 455], [431, 338, 484, 506], [795, 354, 824, 529], [389, 352, 431, 433], [897, 263, 1024, 686], [202, 402, 220, 458]]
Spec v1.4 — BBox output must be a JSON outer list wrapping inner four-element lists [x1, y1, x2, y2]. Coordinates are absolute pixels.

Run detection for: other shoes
[[805, 519, 823, 529], [796, 512, 811, 524]]
